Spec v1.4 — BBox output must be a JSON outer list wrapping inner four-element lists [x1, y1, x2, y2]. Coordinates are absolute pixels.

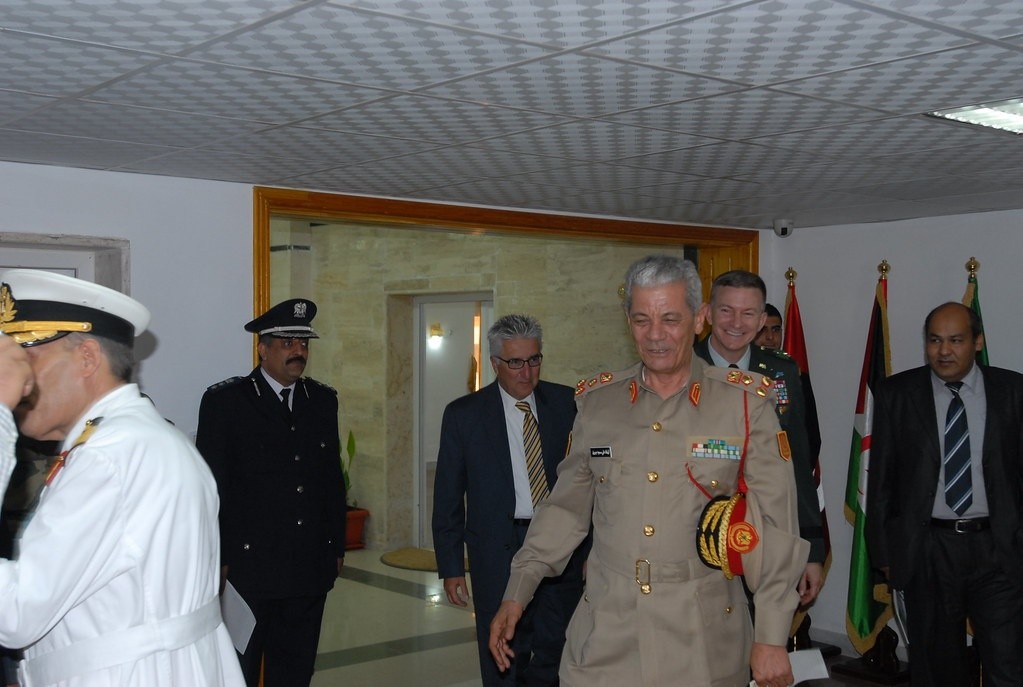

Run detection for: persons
[[196, 299, 346, 687], [489, 253, 810, 687], [693, 269, 832, 653], [864, 300, 1023, 687], [0, 270, 250, 687], [432, 315, 593, 687]]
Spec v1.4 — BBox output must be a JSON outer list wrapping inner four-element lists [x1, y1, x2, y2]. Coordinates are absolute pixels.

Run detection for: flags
[[781, 280, 832, 638], [962, 275, 990, 366], [844, 276, 894, 656]]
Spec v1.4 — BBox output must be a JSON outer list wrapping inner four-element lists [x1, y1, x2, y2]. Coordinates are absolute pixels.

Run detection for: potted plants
[[337, 428, 369, 550]]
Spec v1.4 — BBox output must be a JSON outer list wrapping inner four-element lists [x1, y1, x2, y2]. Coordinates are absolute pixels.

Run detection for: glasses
[[494, 352, 543, 369]]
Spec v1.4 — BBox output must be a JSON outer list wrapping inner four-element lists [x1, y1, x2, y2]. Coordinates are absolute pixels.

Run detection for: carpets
[[380, 546, 469, 573]]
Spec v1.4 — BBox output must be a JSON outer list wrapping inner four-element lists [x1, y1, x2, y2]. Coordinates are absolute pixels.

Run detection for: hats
[[0, 268, 150, 347], [697, 491, 763, 593], [245, 299, 320, 339]]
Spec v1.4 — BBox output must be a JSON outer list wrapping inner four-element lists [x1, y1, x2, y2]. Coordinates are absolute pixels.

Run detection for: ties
[[944, 381, 973, 517], [280, 388, 291, 410], [515, 401, 550, 510]]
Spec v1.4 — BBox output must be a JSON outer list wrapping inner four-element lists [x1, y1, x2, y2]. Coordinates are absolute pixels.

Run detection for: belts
[[514, 518, 531, 526], [931, 518, 990, 533]]
